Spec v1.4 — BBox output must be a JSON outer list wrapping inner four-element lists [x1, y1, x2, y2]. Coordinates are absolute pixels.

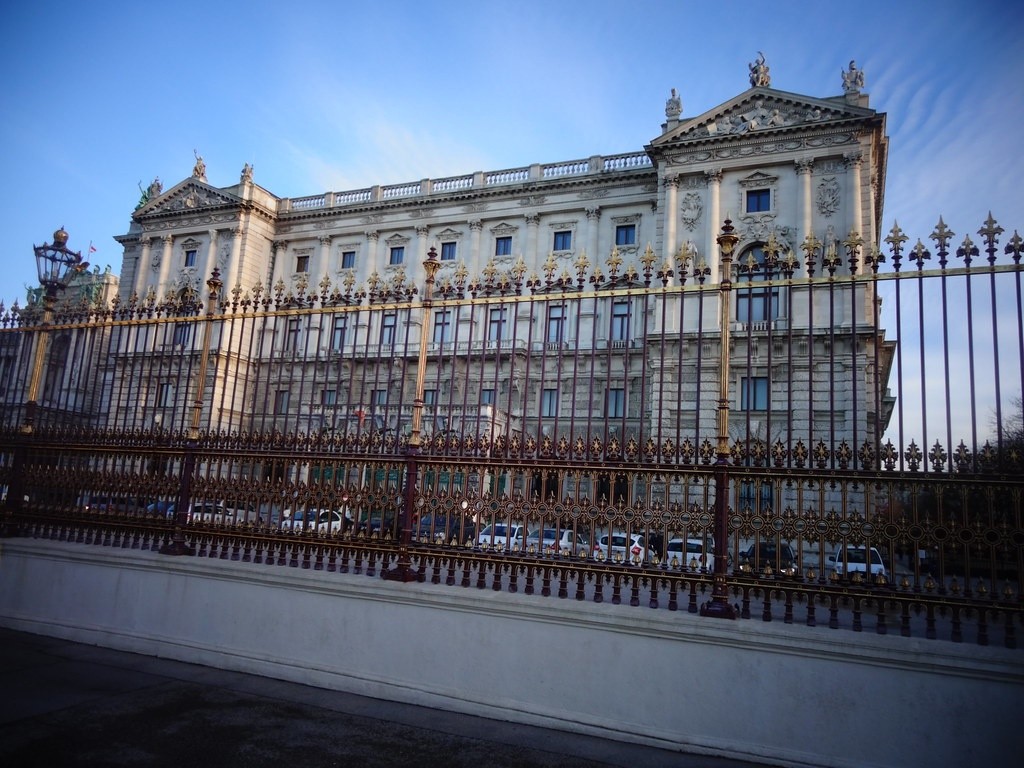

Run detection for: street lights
[[385, 245, 447, 577], [704, 217, 741, 606], [3, 225, 86, 510], [163, 266, 226, 555]]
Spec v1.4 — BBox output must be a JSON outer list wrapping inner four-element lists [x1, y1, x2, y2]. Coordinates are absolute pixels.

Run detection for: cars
[[92, 495, 141, 512], [147, 498, 731, 574], [828, 545, 890, 585], [739, 540, 803, 576]]
